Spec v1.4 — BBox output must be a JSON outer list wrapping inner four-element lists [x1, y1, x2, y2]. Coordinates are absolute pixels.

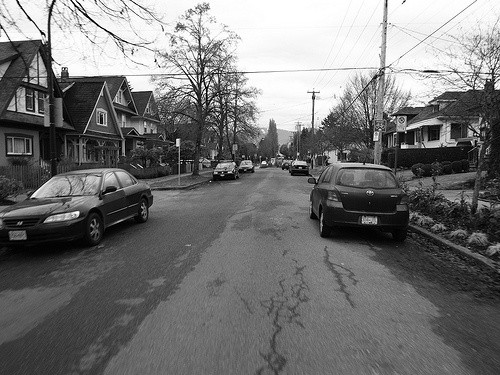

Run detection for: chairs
[[342, 170, 384, 188]]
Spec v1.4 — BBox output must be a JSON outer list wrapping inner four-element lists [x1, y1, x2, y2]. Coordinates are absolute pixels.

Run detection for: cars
[[276, 159, 309, 176], [212, 162, 240, 180], [307, 158, 410, 241], [239, 160, 255, 173], [0, 168, 155, 247]]
